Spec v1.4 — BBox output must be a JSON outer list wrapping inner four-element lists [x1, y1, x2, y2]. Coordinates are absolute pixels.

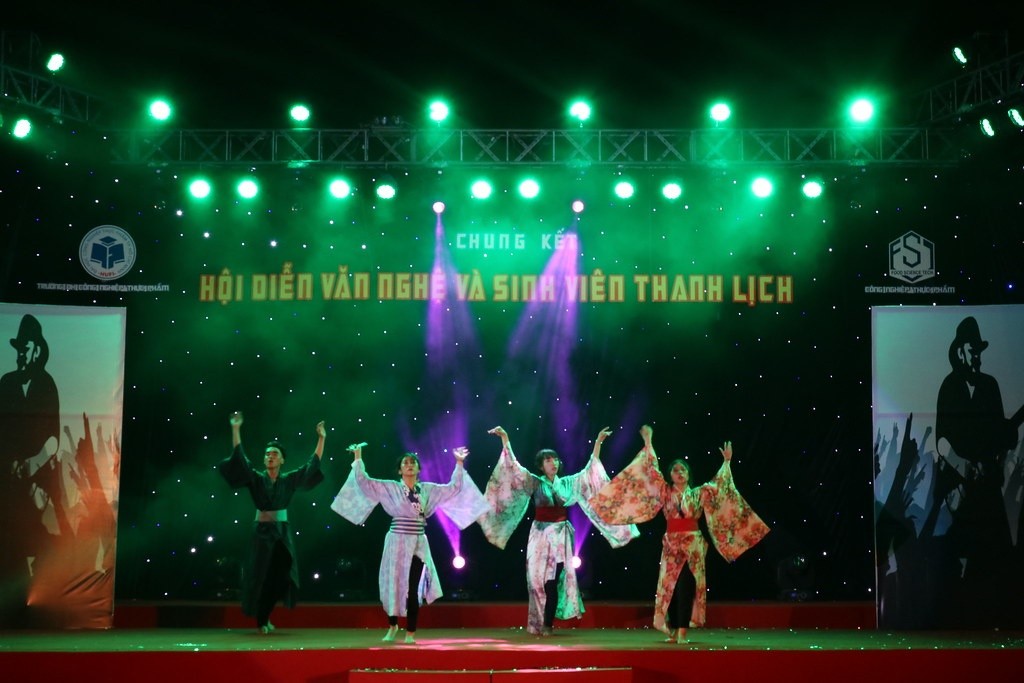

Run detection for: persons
[[480, 425, 642, 638], [217, 410, 327, 636], [330, 441, 482, 644], [595, 425, 770, 645]]
